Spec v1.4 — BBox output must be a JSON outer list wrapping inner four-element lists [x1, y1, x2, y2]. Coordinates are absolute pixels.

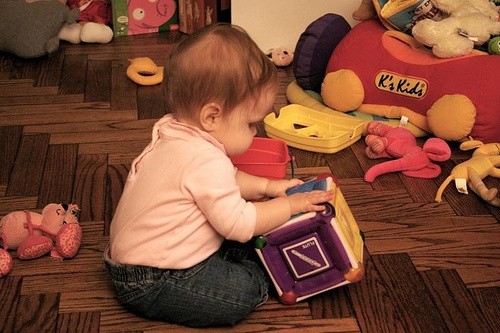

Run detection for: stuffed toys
[[0, 202, 83, 277], [285, 0, 500, 144], [412, 0, 500, 59], [361, 120, 451, 183], [266, 47, 294, 66], [25, 0, 114, 43], [435, 140, 500, 207]]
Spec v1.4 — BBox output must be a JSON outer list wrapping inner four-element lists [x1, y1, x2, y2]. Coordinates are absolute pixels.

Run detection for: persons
[[103, 23, 335, 329]]
